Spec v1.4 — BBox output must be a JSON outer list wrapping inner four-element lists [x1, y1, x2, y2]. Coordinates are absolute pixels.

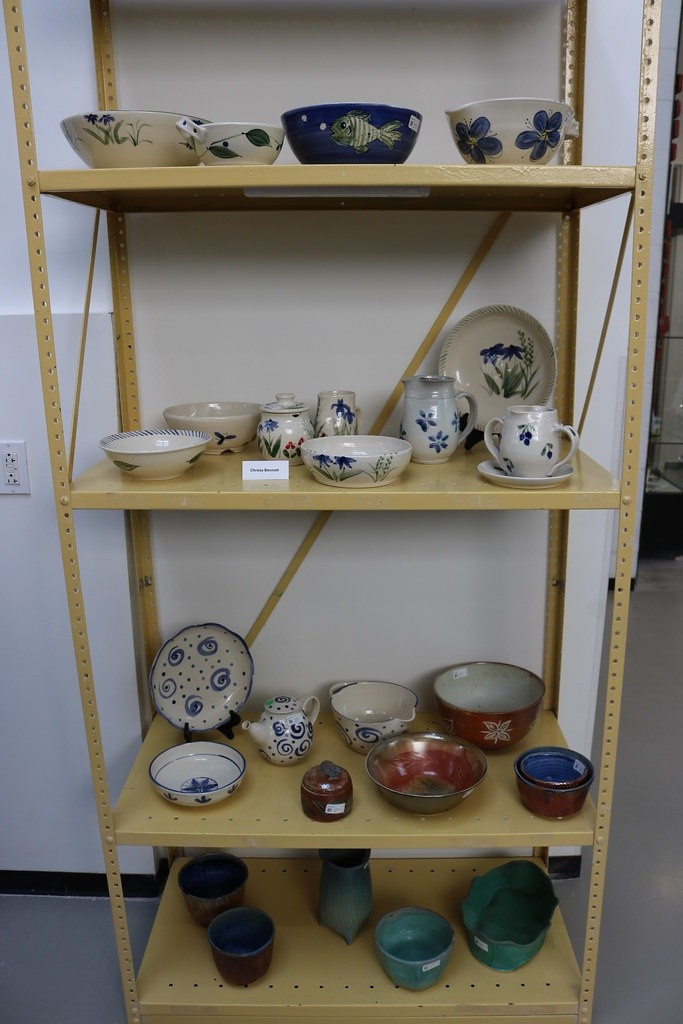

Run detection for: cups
[[484, 405, 579, 478], [300, 760, 354, 823]]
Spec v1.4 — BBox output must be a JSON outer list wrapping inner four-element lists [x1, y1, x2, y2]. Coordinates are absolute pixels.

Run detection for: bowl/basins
[[97, 429, 213, 481], [438, 303, 557, 434], [300, 434, 413, 487], [281, 102, 423, 165], [431, 661, 546, 752], [513, 746, 596, 820], [59, 110, 213, 169], [148, 741, 247, 808], [163, 401, 262, 455], [364, 732, 490, 815], [444, 97, 575, 166], [174, 116, 287, 166], [177, 852, 562, 993]]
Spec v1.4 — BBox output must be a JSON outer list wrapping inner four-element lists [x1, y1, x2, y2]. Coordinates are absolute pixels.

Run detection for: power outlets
[[0, 440, 31, 495]]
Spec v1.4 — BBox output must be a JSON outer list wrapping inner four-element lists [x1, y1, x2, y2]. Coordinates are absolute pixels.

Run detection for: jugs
[[313, 390, 361, 438], [398, 375, 478, 465], [328, 679, 418, 755]]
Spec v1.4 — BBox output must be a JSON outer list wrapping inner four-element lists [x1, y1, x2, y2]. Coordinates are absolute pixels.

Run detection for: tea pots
[[241, 694, 321, 767]]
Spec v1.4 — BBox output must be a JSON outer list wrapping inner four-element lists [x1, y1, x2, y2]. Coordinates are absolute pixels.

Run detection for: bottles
[[256, 393, 313, 466]]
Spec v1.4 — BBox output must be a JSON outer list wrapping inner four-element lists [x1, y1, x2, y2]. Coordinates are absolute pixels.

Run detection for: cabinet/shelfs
[[3, 0, 660, 1024]]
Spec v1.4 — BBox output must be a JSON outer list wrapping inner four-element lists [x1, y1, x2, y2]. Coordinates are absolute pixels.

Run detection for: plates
[[148, 622, 256, 734], [477, 458, 575, 490]]
